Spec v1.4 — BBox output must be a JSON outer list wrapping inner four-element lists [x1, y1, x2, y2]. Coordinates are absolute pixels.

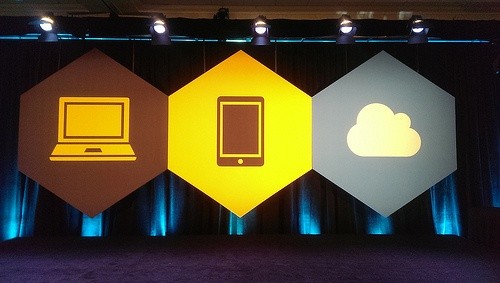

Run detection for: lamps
[[26, 7, 435, 45]]
